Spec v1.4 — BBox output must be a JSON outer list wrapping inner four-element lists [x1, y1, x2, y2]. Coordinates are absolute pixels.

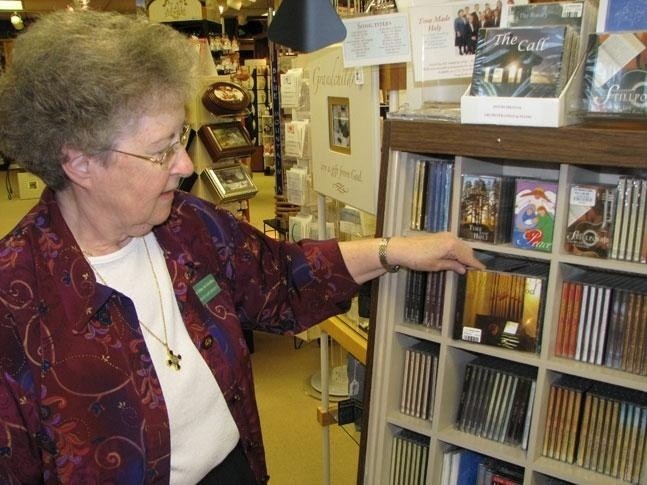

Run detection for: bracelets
[[379, 237, 400, 273]]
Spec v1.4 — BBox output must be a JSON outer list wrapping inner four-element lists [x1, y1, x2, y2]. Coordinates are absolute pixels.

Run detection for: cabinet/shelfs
[[262, 11, 646, 485]]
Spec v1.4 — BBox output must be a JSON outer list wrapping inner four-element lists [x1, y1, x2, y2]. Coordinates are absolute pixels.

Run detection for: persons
[[454, 0, 515, 55], [0, 0, 486, 485]]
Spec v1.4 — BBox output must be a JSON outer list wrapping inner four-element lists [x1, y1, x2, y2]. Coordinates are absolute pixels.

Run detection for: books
[[390, 428, 431, 484], [440, 445, 524, 485], [411, 159, 453, 233], [458, 176, 647, 264], [541, 376, 647, 485], [454, 357, 539, 450], [470, 0, 647, 120], [555, 271, 647, 377], [401, 341, 440, 422], [404, 257, 550, 340]]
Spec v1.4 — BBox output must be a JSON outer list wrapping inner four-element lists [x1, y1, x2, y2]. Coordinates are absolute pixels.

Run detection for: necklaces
[[84, 236, 182, 370]]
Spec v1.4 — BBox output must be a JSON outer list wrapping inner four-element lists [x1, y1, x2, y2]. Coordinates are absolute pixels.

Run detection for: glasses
[[98, 122, 192, 166]]
